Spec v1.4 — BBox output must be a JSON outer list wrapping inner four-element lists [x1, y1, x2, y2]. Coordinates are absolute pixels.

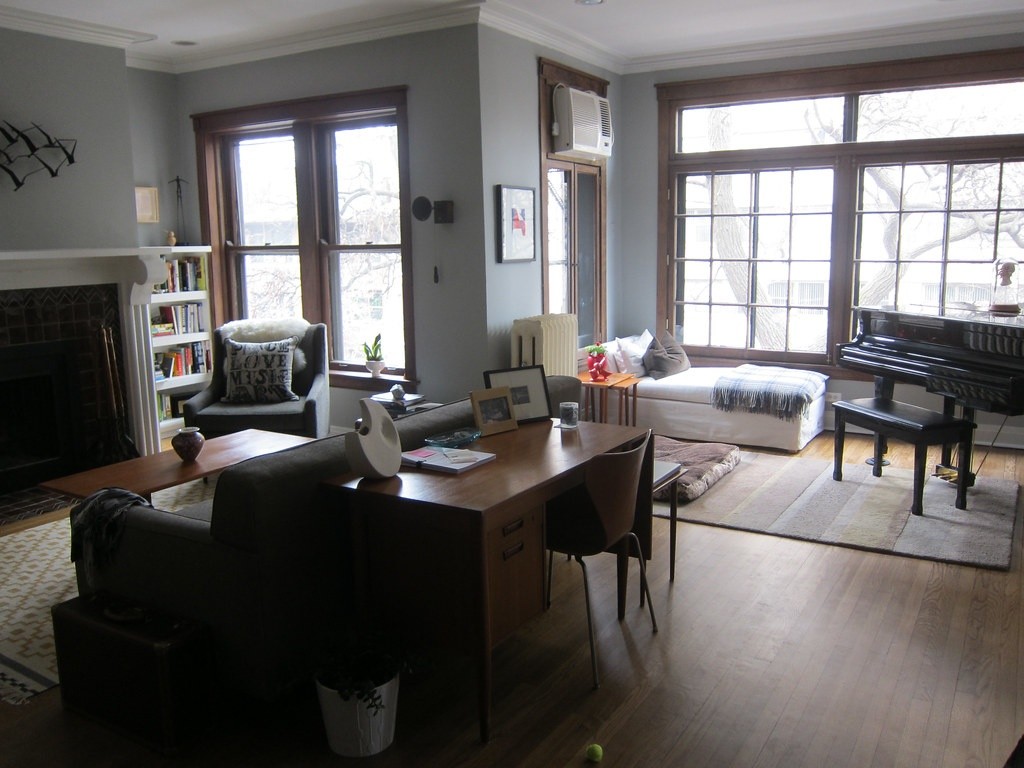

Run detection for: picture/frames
[[466, 386, 519, 438], [495, 184, 537, 263], [482, 364, 553, 424]]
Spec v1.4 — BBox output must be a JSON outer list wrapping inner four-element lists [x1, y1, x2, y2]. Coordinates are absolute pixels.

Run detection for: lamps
[[409, 193, 455, 226]]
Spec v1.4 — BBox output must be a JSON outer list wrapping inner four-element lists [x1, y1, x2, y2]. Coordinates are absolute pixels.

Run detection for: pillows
[[643, 332, 691, 379], [220, 335, 301, 403], [613, 348, 627, 374], [616, 327, 655, 379]]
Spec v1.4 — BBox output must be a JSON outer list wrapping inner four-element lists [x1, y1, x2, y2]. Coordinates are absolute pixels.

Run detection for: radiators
[[509, 310, 581, 378]]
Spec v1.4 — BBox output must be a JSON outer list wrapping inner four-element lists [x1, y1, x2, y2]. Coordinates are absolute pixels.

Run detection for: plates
[[423, 428, 480, 447]]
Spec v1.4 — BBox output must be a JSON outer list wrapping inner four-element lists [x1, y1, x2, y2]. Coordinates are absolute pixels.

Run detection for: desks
[[319, 419, 661, 744], [623, 460, 688, 607]]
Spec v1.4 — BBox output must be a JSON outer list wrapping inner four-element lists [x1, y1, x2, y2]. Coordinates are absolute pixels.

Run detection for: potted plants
[[363, 333, 387, 377]]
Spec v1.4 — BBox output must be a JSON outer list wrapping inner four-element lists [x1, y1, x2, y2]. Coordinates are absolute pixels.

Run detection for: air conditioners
[[552, 83, 614, 162]]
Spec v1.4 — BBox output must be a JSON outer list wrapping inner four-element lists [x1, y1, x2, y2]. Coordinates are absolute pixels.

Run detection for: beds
[[575, 331, 827, 456]]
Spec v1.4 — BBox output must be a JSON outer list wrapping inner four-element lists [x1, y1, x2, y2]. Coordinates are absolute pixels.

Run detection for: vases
[[170, 426, 206, 464]]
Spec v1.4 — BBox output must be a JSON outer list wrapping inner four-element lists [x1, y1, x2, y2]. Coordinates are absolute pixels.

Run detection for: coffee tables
[[41, 427, 319, 519]]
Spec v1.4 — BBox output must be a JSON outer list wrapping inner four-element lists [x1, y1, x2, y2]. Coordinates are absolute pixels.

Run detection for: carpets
[[645, 448, 1020, 571], [0, 471, 220, 707]]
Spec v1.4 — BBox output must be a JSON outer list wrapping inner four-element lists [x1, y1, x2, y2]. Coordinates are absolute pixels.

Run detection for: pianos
[[832, 305, 1024, 467]]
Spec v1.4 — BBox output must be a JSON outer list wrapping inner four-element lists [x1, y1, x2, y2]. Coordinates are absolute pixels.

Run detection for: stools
[[831, 397, 977, 515]]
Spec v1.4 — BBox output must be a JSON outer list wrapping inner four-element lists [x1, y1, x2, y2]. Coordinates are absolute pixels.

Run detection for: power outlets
[[825, 392, 842, 403]]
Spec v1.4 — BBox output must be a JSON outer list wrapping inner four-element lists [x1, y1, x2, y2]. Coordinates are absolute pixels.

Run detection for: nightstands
[[578, 369, 643, 425]]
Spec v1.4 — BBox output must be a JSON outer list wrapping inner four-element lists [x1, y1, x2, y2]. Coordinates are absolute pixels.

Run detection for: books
[[151, 302, 204, 336], [400, 446, 497, 475], [154, 255, 207, 294], [153, 340, 212, 383], [156, 393, 172, 423]]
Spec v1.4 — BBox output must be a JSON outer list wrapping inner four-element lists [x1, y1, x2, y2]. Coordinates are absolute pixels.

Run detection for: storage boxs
[[46, 592, 220, 760]]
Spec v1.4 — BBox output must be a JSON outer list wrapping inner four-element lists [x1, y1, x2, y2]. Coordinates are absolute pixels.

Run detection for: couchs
[[179, 320, 336, 439], [82, 370, 583, 704]]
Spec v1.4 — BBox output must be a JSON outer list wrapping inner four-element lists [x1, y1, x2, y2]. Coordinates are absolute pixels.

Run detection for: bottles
[[170, 426, 204, 463], [989, 262, 1020, 313]]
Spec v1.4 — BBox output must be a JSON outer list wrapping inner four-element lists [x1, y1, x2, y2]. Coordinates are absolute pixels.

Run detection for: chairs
[[542, 427, 660, 691]]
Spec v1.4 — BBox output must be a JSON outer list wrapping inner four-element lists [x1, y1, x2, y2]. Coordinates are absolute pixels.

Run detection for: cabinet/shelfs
[[142, 240, 220, 438]]
